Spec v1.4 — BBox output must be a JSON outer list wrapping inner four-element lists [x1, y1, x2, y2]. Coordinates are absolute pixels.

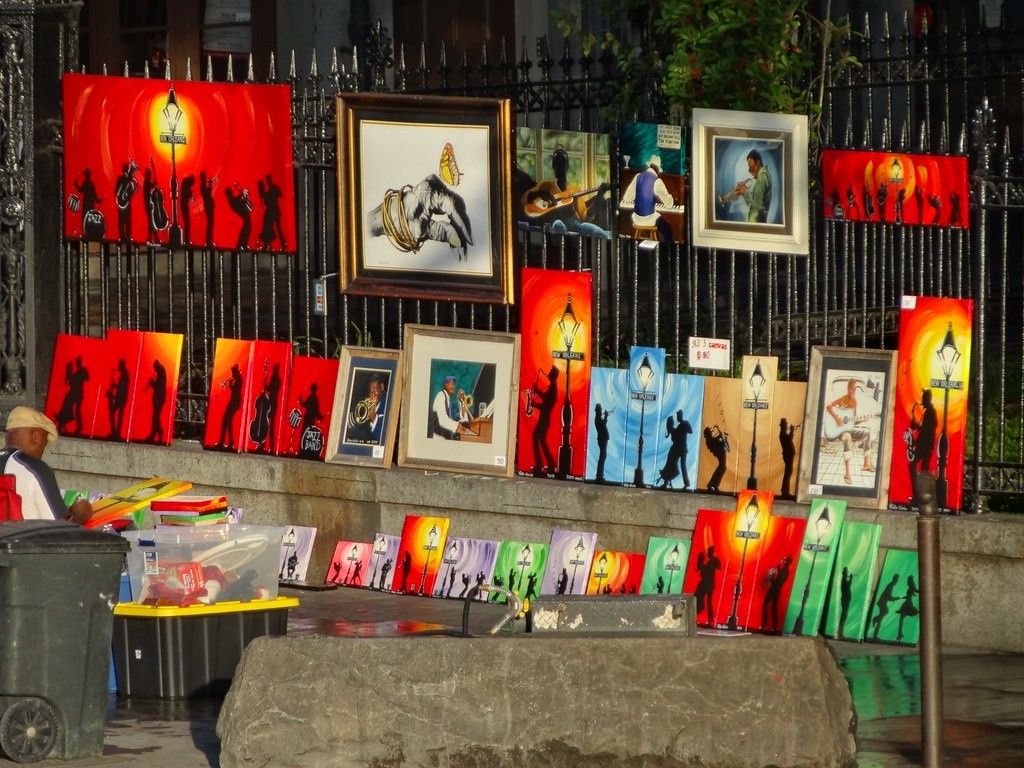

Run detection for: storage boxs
[[111, 596, 300, 700], [120, 524, 288, 607], [108, 571, 132, 694]]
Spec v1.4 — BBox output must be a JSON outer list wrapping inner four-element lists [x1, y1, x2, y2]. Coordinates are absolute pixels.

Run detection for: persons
[[348, 373, 387, 444], [0, 406, 92, 526]]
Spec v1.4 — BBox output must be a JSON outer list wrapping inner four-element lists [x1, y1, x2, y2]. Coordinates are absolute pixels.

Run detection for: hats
[[5, 406, 58, 443]]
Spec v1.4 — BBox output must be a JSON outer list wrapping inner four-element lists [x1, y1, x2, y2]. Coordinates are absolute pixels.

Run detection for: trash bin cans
[[0, 518, 132, 763]]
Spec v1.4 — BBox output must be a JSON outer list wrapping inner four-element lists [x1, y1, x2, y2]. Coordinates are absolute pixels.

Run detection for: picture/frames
[[795, 345, 898, 509], [335, 93, 515, 306], [398, 321, 521, 478], [324, 345, 404, 470], [692, 107, 811, 255]]
